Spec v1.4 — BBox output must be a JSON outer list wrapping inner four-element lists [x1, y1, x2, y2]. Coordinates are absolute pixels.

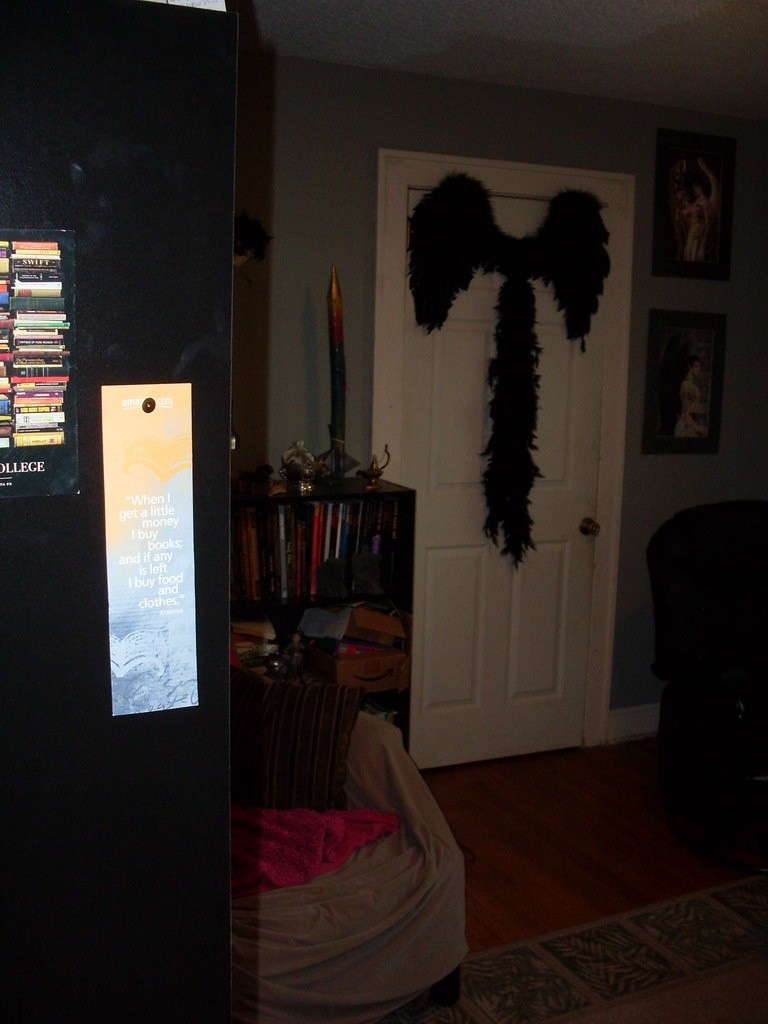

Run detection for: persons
[[283, 631, 306, 684]]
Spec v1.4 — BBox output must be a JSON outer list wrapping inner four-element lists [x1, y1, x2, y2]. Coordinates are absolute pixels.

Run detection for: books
[[229, 608, 280, 676], [0, 240, 69, 449], [230, 498, 398, 603]]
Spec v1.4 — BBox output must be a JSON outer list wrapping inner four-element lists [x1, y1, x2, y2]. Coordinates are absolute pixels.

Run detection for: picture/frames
[[639, 308, 728, 455], [650, 126, 740, 280]]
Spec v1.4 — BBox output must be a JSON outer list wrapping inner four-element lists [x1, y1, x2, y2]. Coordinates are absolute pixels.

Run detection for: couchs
[[648, 500, 768, 875]]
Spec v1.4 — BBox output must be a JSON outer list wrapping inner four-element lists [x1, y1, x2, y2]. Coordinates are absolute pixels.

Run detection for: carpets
[[380, 875, 768, 1024]]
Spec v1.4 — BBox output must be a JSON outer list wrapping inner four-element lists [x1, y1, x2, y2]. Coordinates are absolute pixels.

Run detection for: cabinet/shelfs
[[231, 476, 416, 754]]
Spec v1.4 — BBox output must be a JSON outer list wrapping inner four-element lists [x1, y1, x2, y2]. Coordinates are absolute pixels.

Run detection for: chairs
[[231, 710, 466, 1024]]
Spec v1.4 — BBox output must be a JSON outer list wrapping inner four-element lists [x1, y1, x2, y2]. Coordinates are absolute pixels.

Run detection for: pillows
[[228, 673, 362, 812]]
